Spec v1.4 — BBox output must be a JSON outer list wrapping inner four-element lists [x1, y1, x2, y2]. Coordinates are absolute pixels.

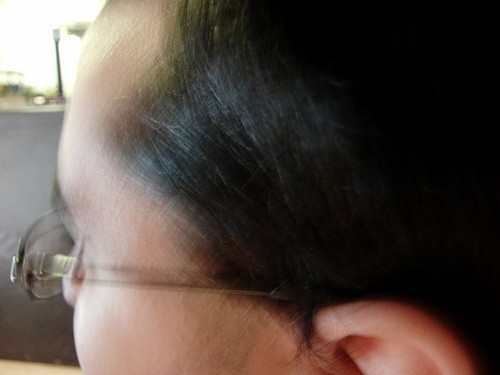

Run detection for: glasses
[[7, 209, 355, 308]]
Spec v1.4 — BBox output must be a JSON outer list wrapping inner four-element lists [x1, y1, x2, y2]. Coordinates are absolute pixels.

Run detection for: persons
[[7, 0, 499, 375]]
